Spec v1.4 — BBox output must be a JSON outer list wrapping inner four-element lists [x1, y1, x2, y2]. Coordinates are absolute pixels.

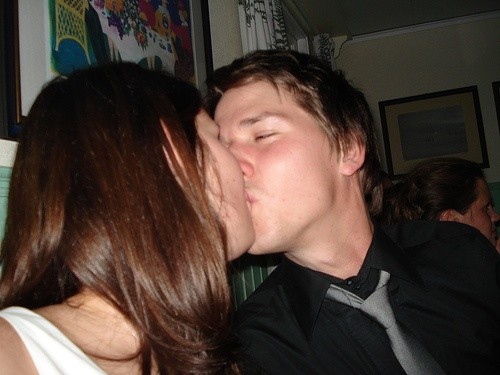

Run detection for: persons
[[0, 62, 256, 375], [201, 48, 500, 374]]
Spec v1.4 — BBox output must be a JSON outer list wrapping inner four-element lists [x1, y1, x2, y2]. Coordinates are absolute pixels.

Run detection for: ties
[[324, 270, 446, 375]]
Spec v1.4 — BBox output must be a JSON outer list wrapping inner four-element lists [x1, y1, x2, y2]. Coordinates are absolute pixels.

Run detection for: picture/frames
[[0, 0, 216, 145], [377, 85, 491, 181]]
